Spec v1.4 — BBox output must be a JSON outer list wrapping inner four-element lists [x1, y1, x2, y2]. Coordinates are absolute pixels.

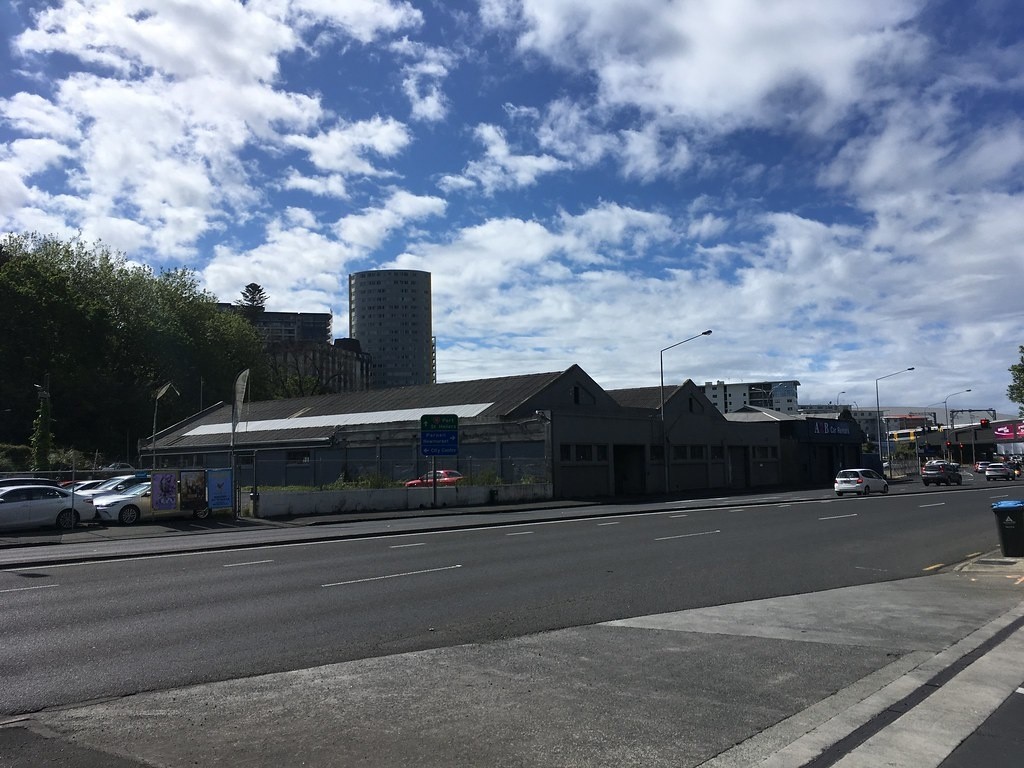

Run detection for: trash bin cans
[[990, 500, 1024, 558]]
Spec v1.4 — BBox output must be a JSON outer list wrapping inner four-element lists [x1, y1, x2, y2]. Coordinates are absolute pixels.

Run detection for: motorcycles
[[1014, 467, 1021, 477]]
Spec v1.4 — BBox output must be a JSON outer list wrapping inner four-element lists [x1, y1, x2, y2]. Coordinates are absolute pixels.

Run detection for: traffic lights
[[981, 420, 985, 425], [947, 442, 950, 446], [960, 444, 963, 448]]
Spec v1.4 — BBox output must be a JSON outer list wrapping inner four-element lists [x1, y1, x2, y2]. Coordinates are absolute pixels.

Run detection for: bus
[[92, 481, 212, 526]]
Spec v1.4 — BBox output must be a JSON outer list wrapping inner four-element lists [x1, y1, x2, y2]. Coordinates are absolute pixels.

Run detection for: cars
[[109, 462, 134, 470], [921, 460, 962, 486], [882, 459, 893, 470], [0, 485, 96, 531], [1009, 455, 1022, 463], [405, 470, 463, 487], [60, 474, 152, 493], [834, 469, 888, 496], [0, 478, 58, 488], [977, 462, 991, 473], [975, 462, 980, 471], [985, 462, 1015, 481]]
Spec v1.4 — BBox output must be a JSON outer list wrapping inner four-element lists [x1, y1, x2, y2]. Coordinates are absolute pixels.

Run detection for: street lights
[[876, 367, 915, 459], [852, 401, 860, 425], [882, 418, 892, 479], [945, 389, 972, 461], [924, 401, 946, 460], [660, 330, 713, 496], [752, 383, 787, 410], [837, 392, 845, 405]]
